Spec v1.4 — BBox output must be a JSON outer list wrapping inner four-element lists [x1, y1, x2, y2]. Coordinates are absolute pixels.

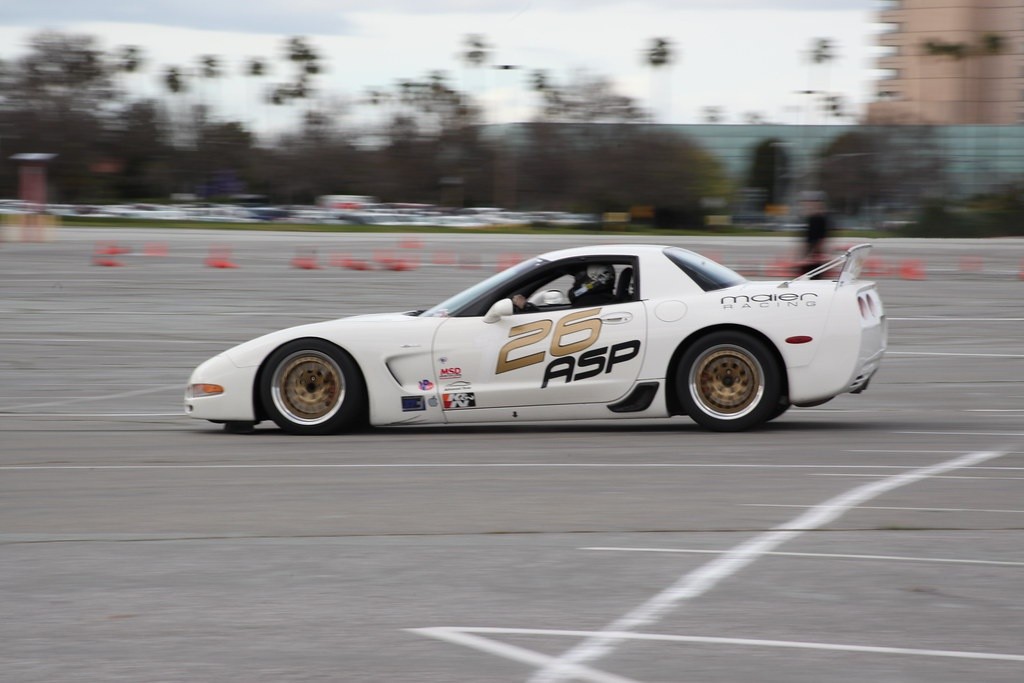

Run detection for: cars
[[184, 243, 887, 435]]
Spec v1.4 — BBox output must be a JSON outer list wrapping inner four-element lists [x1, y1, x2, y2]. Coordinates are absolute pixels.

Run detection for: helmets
[[567, 263, 616, 301]]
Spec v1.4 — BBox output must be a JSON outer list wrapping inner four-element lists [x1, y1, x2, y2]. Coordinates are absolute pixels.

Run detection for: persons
[[513, 264, 615, 314]]
